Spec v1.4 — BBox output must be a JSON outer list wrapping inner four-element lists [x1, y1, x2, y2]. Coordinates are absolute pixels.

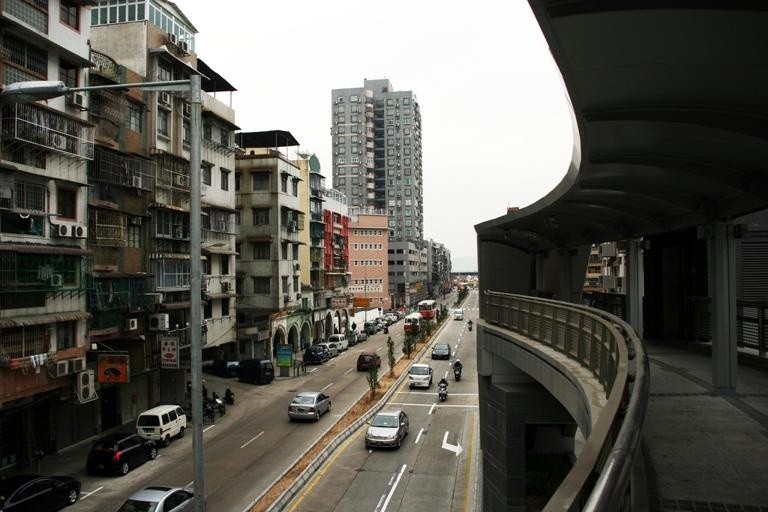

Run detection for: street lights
[[0, 72, 208, 512]]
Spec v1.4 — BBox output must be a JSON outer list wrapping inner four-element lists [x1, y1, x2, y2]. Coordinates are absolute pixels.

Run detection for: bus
[[404, 312, 423, 335], [417, 299, 437, 320]]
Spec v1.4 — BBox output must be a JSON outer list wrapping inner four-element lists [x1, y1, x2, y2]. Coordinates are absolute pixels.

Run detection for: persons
[[185, 377, 235, 423], [452, 359, 463, 373], [365, 327, 370, 337], [467, 319, 473, 325], [351, 321, 357, 331], [437, 376, 449, 394], [385, 321, 389, 333]]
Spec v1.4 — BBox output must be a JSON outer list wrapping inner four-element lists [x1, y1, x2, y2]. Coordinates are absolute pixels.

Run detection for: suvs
[[357, 351, 381, 371]]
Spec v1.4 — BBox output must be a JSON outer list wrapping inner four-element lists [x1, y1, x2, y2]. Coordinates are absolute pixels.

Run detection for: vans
[[213, 359, 240, 378], [327, 333, 349, 352], [135, 404, 187, 448], [237, 359, 274, 385], [318, 343, 338, 359]]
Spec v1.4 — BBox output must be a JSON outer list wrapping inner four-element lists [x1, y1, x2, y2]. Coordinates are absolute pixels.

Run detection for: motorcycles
[[225, 394, 236, 405], [437, 384, 449, 403], [452, 366, 463, 382], [468, 323, 473, 332], [208, 398, 224, 409]]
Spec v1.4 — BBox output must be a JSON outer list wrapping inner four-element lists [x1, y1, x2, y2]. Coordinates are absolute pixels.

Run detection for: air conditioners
[[47, 355, 97, 404], [51, 132, 68, 153], [130, 175, 143, 187], [49, 273, 63, 288], [70, 91, 89, 110], [49, 223, 88, 239], [283, 220, 302, 302], [148, 314, 170, 332], [158, 93, 235, 302], [124, 317, 138, 331]]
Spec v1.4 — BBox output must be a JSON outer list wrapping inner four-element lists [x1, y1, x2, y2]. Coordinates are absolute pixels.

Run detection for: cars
[[288, 392, 332, 422], [432, 341, 451, 360], [303, 346, 329, 365], [114, 485, 196, 512], [364, 408, 409, 450], [85, 432, 159, 477], [345, 305, 409, 346], [453, 309, 464, 320], [0, 475, 83, 511]]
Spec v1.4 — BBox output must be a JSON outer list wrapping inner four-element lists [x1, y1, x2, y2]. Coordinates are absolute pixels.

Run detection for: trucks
[[407, 364, 433, 389]]
